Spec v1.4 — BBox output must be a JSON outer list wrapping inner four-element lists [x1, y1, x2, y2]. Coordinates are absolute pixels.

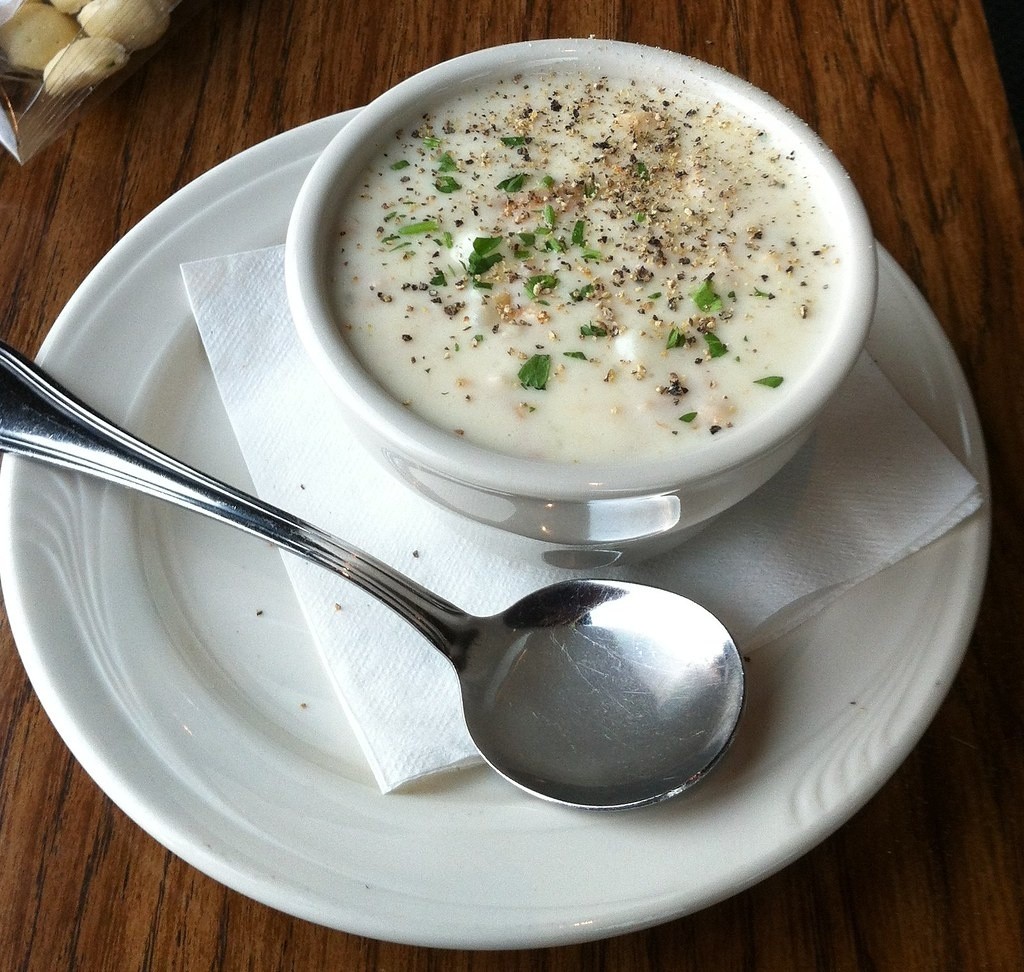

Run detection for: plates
[[0, 106, 991, 951]]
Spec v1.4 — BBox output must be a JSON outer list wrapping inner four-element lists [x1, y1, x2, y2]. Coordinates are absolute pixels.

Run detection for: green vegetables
[[388, 130, 785, 422]]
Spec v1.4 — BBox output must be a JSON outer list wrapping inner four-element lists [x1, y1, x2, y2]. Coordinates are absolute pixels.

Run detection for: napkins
[[180, 250, 982, 801]]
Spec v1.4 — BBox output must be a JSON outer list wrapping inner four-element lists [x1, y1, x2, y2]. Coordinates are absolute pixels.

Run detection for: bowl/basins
[[285, 37, 877, 547]]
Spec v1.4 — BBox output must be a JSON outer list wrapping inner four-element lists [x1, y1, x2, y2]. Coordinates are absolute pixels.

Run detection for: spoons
[[0, 343, 748, 813]]
